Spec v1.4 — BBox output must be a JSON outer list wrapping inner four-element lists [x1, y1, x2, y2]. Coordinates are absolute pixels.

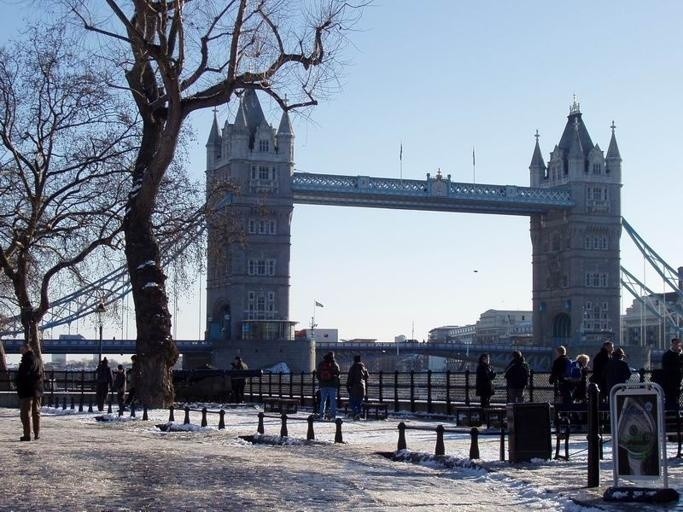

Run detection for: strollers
[[305, 383, 337, 422]]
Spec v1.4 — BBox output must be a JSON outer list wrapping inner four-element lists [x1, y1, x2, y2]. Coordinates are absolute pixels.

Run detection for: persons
[[317, 352, 340, 422], [125, 355, 138, 409], [230, 363, 237, 403], [476, 339, 683, 424], [235, 357, 248, 403], [346, 356, 369, 419], [97, 360, 112, 411], [112, 365, 125, 411], [15, 345, 44, 441]]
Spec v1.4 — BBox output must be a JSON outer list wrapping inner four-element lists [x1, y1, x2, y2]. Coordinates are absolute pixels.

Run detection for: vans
[[59, 334, 87, 345]]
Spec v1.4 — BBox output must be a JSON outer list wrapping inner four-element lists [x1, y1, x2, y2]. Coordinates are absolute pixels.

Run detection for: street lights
[[95, 291, 110, 364]]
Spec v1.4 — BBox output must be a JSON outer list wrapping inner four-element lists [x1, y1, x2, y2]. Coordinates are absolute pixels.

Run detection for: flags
[[316, 302, 323, 307]]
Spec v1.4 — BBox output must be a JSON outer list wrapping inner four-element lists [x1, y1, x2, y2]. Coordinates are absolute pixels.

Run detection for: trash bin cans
[[505, 401, 552, 464]]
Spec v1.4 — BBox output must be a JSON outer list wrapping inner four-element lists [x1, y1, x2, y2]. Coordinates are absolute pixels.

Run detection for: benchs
[[454, 406, 506, 427], [262, 397, 299, 414], [344, 401, 388, 420]]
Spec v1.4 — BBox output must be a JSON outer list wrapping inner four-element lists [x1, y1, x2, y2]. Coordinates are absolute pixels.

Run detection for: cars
[[626, 365, 640, 388]]
